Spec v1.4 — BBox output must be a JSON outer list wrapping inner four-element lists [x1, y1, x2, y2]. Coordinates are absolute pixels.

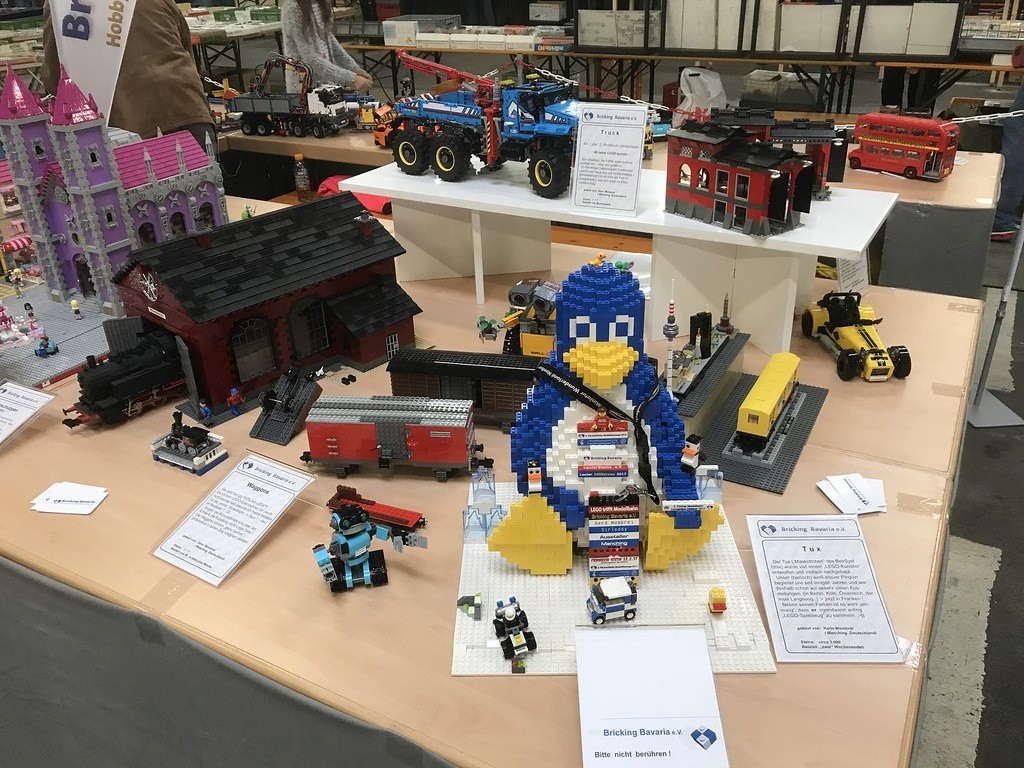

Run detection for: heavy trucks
[[232, 55, 349, 139], [391, 71, 606, 200]]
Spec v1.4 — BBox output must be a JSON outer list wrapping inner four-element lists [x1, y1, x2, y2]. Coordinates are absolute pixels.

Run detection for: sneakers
[[990, 217, 1022, 241]]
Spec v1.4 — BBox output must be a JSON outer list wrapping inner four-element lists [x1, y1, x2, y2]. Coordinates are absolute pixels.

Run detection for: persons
[[280, 0, 372, 93], [38, 0, 219, 169]]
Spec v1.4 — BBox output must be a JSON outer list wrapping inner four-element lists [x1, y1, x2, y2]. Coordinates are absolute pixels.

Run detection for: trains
[[60, 328, 190, 428]]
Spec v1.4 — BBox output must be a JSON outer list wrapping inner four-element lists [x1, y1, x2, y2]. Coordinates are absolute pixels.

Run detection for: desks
[[2, 187, 991, 768], [231, 97, 1006, 300], [336, 156, 903, 305]]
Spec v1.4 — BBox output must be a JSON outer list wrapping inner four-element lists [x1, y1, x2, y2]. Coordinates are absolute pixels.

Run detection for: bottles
[[294, 152, 313, 203]]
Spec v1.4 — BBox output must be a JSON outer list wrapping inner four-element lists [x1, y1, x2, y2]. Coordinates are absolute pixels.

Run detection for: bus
[[846, 111, 959, 181]]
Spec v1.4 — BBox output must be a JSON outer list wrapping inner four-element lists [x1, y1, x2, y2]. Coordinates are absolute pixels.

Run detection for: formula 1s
[[802, 290, 911, 382]]
